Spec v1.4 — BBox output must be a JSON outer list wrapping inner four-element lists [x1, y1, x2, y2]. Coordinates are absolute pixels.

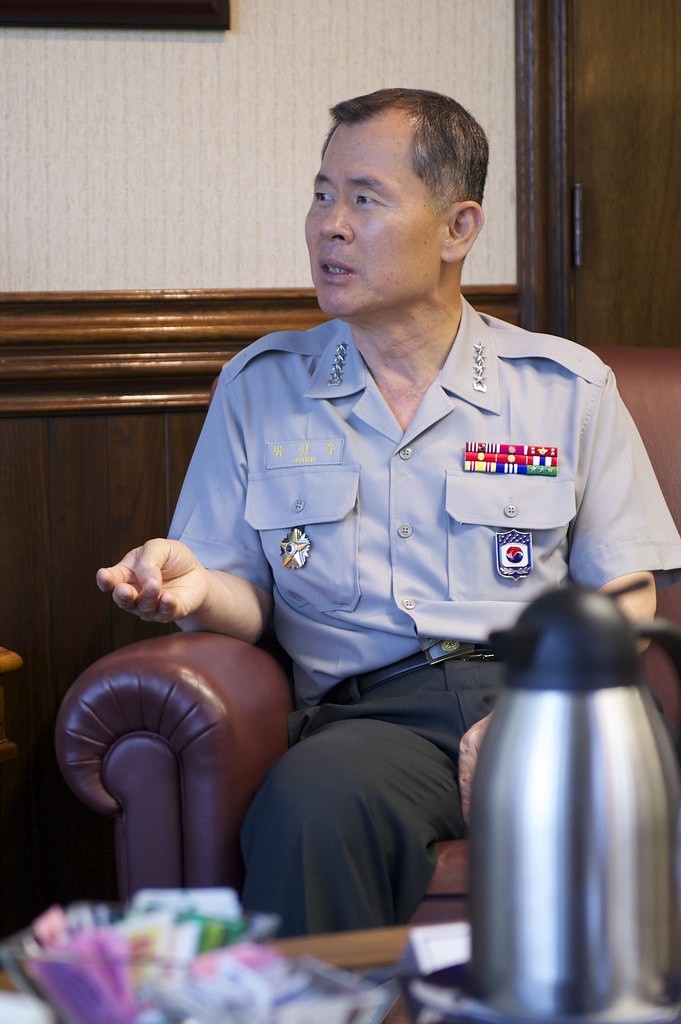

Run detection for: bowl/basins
[[13, 908, 281, 1024]]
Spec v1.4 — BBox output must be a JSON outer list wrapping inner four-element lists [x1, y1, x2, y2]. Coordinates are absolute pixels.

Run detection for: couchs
[[52, 347, 681, 949]]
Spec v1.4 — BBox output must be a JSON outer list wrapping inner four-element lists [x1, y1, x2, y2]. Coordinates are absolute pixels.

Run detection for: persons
[[96, 87, 681, 945]]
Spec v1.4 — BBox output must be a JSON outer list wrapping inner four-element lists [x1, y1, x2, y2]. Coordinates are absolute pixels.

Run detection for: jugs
[[467, 581, 681, 1023]]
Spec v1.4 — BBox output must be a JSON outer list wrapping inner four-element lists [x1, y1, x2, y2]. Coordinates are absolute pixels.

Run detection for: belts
[[313, 639, 506, 704]]
